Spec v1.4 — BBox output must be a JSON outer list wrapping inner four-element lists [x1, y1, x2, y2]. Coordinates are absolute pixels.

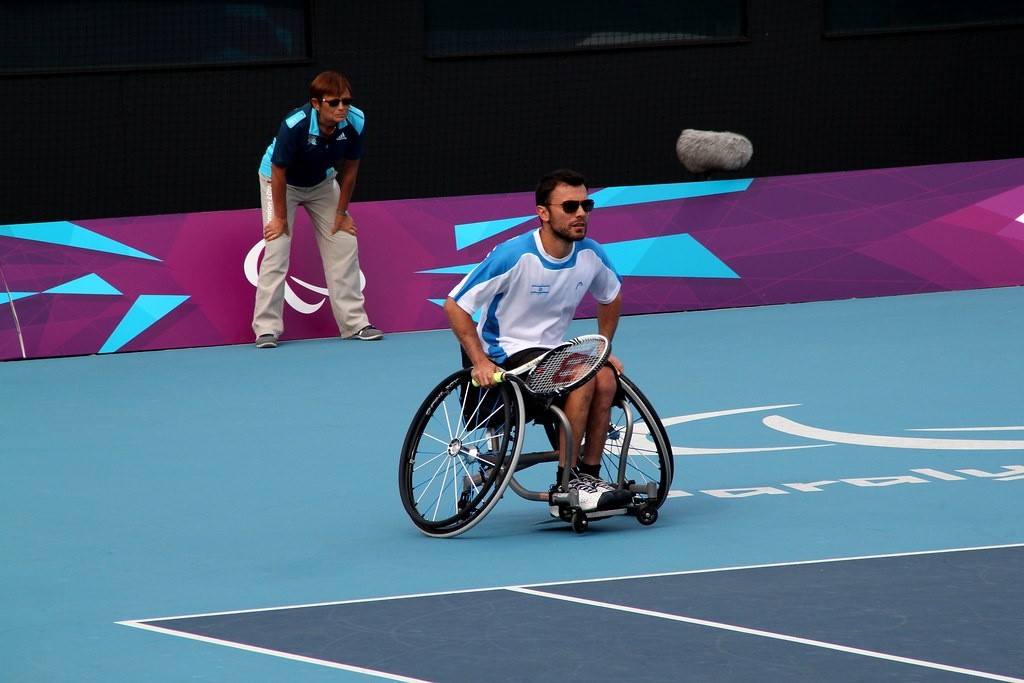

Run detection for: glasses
[[544, 199, 594, 213], [319, 98, 352, 107]]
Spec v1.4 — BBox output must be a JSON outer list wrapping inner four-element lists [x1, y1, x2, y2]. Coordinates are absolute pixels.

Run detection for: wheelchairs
[[398, 341, 674, 539]]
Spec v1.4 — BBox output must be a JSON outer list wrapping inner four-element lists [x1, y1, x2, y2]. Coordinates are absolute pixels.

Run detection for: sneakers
[[549, 478, 615, 518], [256, 334, 277, 347], [578, 473, 632, 507], [344, 325, 384, 340]]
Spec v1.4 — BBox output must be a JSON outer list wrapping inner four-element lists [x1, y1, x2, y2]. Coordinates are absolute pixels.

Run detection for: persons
[[251, 70, 384, 348], [444, 170, 633, 517]]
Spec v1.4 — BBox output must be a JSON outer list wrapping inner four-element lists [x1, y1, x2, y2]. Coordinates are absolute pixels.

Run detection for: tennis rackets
[[469, 331, 612, 402]]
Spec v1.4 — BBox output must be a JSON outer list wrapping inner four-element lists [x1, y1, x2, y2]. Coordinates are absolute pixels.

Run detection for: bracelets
[[336, 208, 349, 216]]
